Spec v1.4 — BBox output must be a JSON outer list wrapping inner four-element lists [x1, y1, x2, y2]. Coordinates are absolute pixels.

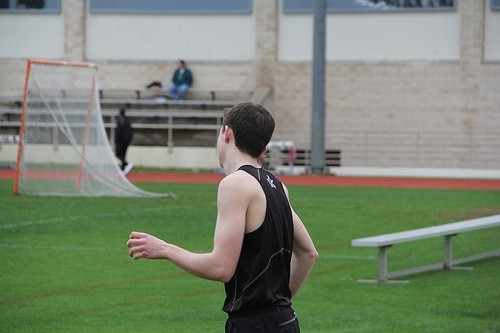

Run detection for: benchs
[[351, 213, 500, 284], [259, 149, 342, 167], [0, 83, 254, 145]]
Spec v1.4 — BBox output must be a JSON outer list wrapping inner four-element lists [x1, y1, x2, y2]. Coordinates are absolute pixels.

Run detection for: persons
[[168, 59, 194, 100], [125, 102, 317, 331], [111, 107, 134, 176]]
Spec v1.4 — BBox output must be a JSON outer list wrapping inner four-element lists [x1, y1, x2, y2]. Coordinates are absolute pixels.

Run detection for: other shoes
[[123, 163, 132, 176]]
